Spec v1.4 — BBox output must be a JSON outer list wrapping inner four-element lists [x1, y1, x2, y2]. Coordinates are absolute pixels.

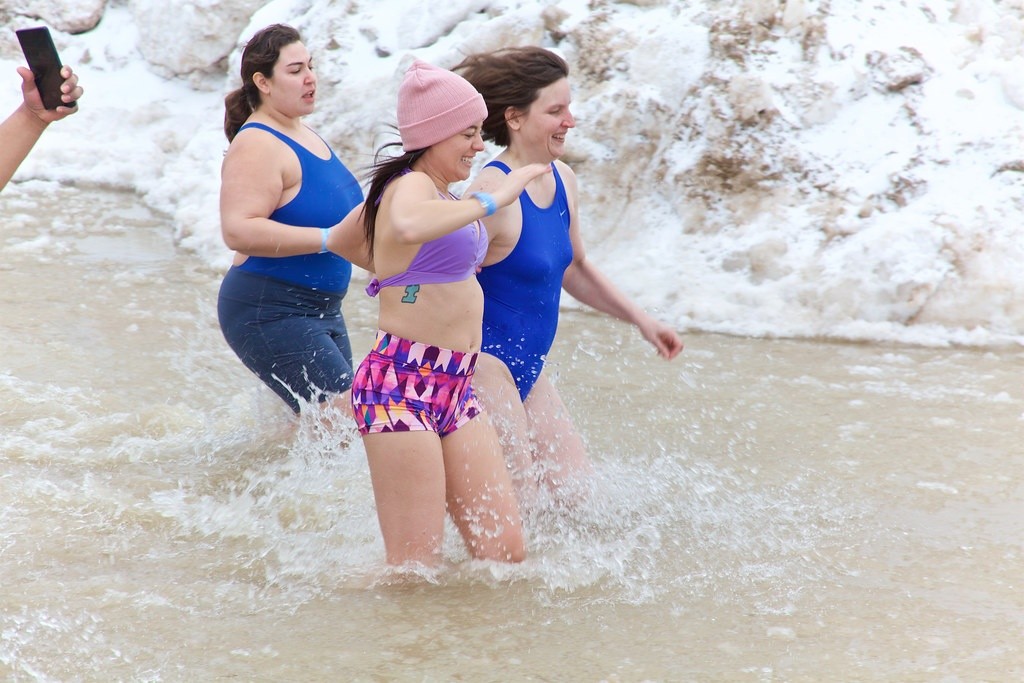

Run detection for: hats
[[397, 59, 488, 151]]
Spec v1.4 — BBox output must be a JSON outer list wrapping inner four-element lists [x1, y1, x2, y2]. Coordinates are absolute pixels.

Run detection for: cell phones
[[15, 26, 76, 110]]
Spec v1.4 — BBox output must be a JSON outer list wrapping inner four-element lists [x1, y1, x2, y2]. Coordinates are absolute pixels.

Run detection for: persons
[[324, 58, 555, 576], [451, 46, 686, 515], [0, 63, 85, 192], [215, 22, 376, 448]]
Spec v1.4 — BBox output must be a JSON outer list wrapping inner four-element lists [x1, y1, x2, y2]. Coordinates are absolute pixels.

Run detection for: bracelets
[[469, 191, 496, 217]]
